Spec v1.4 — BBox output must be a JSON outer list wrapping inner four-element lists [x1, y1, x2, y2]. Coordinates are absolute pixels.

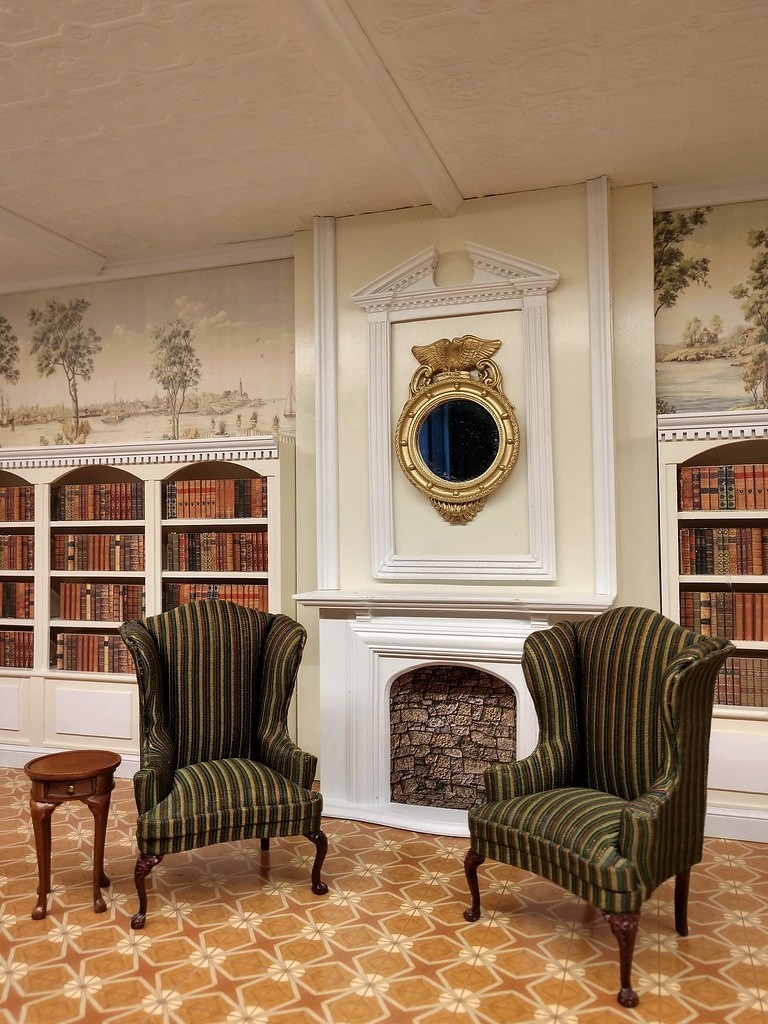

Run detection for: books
[[678, 462, 768, 707], [0, 476, 268, 674]]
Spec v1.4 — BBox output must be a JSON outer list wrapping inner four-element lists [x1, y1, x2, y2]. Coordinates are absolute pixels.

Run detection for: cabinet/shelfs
[[658, 410, 768, 843], [0, 433, 295, 781]]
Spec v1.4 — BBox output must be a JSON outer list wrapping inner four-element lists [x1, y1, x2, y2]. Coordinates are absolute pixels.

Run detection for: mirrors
[[395, 334, 519, 533]]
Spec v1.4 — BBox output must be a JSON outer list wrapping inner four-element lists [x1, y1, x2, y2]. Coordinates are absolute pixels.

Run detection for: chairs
[[116, 601, 329, 930], [464, 605, 736, 1006]]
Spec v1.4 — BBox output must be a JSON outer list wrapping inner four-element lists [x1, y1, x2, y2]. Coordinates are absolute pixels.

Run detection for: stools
[[24, 750, 120, 926]]
